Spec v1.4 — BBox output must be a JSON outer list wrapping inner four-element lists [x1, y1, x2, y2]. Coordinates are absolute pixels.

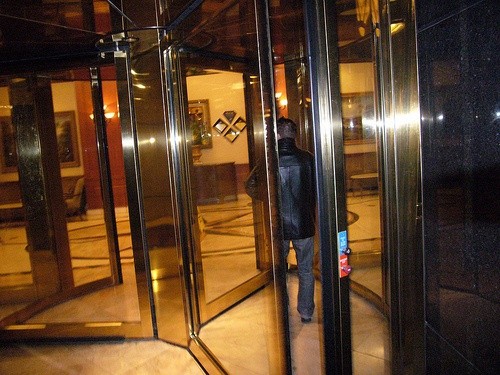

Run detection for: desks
[[349, 172, 378, 197]]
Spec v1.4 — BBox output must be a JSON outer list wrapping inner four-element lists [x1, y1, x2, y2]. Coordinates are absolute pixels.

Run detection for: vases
[[192, 146, 203, 166]]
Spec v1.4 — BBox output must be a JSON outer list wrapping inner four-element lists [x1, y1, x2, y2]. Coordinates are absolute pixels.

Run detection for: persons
[[243, 117, 318, 323]]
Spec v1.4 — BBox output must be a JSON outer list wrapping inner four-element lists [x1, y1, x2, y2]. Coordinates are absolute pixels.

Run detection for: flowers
[[189, 106, 208, 144]]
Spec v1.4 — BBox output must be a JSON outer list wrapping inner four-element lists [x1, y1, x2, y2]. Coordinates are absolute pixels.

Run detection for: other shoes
[[301, 317, 312, 323]]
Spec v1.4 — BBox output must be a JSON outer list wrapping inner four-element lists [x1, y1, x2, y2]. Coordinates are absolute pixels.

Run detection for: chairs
[[65, 178, 87, 221]]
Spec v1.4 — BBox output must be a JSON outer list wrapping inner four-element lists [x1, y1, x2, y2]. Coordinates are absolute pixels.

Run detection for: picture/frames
[[188, 99, 213, 149], [0, 111, 80, 173], [342, 90, 377, 145]]
